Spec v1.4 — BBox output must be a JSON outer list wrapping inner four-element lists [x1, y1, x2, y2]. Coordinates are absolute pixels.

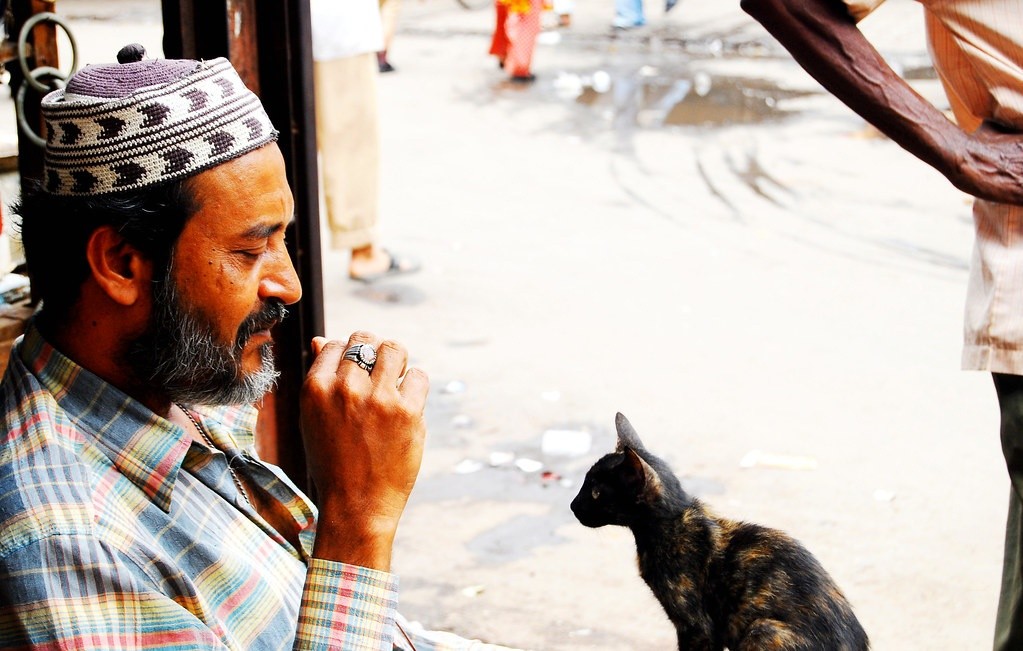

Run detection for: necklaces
[[174, 401, 250, 503]]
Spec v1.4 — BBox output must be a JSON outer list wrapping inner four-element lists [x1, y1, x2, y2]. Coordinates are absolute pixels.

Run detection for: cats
[[568, 411, 871, 651]]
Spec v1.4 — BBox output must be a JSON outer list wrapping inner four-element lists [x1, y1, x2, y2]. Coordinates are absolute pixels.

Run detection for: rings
[[341, 344, 377, 370]]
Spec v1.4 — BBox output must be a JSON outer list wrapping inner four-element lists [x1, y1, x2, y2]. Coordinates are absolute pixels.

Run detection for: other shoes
[[375, 49, 392, 72], [348, 250, 419, 281], [511, 73, 537, 84]]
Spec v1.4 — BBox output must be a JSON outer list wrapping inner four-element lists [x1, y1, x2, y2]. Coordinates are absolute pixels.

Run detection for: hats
[[41, 45, 277, 198]]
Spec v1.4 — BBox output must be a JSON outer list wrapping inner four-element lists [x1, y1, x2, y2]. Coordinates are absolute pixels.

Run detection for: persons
[[1, 42, 529, 651], [489, 0, 571, 81], [612, 0, 678, 27], [739, 0, 1023, 650], [310, 0, 417, 279]]
